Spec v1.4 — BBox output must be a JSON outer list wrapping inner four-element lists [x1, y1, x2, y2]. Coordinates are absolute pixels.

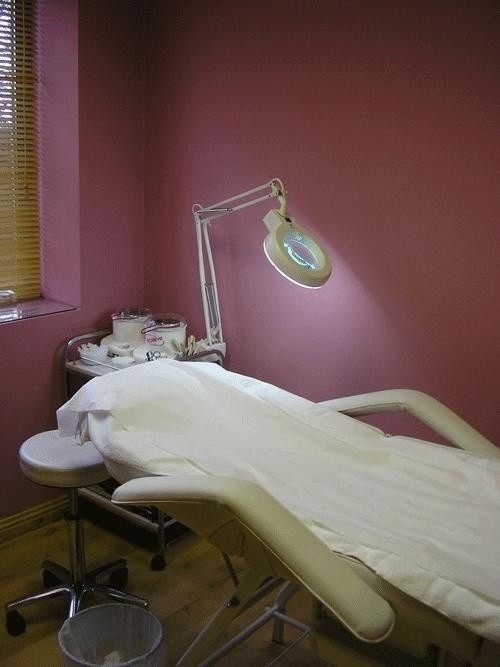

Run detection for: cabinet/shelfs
[[59, 329, 226, 551]]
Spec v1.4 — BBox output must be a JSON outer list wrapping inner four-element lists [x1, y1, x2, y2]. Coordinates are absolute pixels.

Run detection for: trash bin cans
[[58, 603, 163, 666]]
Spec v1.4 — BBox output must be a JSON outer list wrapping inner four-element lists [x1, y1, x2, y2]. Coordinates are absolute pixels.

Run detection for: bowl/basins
[[79, 346, 108, 366], [111, 356, 134, 370]]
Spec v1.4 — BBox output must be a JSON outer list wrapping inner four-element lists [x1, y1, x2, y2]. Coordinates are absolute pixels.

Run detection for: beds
[[66, 354, 499, 666]]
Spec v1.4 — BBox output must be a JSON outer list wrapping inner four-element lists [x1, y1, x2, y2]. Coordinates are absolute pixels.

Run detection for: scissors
[[146, 350, 160, 360]]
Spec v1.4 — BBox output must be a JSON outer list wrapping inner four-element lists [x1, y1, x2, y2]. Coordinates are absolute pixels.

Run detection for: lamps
[[192, 178, 331, 365]]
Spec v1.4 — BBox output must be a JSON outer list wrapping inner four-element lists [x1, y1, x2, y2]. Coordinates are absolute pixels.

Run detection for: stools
[[5, 429, 149, 638]]
